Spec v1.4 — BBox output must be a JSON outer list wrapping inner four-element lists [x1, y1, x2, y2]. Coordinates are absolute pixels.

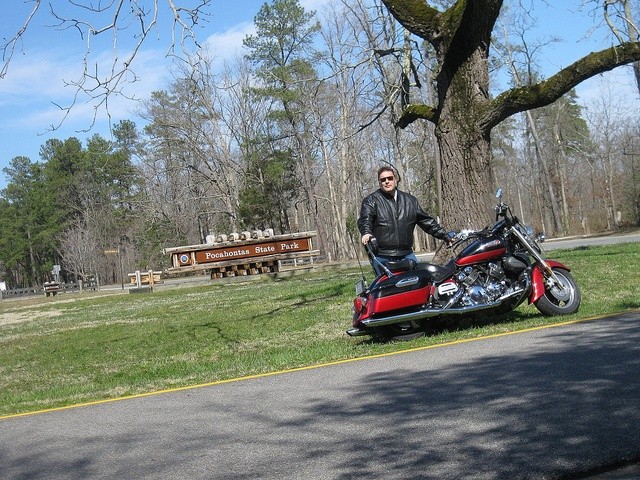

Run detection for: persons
[[357, 166, 456, 279]]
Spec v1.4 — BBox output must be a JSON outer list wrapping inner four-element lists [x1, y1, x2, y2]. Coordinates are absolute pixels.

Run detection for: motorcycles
[[346, 188, 581, 342]]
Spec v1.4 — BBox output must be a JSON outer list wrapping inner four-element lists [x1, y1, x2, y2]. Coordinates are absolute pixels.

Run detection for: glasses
[[379, 176, 394, 181]]
